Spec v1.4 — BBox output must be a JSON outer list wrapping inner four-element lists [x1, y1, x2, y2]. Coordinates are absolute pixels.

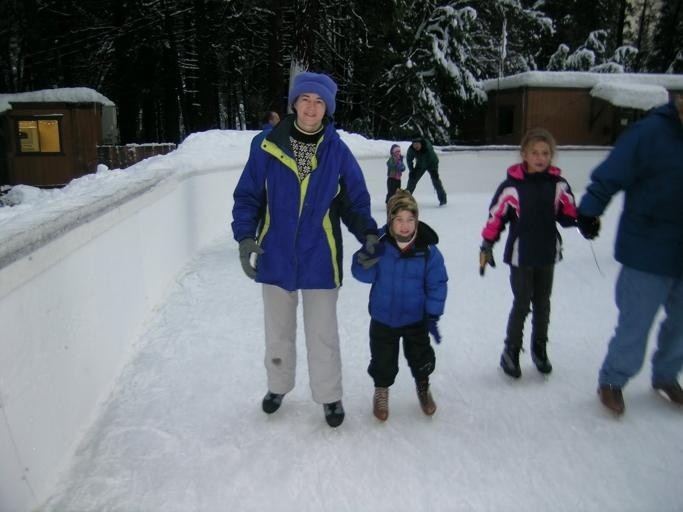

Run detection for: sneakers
[[532, 351, 552, 374], [373, 387, 389, 420], [597, 383, 625, 411], [654, 379, 683, 404], [416, 378, 436, 414], [500, 351, 521, 378], [263, 391, 285, 413], [323, 400, 344, 427], [440, 200, 446, 205]]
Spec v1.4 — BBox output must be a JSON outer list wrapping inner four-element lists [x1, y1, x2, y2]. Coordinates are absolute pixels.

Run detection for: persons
[[232, 71, 378, 427], [577, 55, 683, 414], [386, 144, 406, 206], [480, 126, 603, 377], [352, 190, 449, 419], [249, 112, 281, 157], [402, 139, 447, 205]]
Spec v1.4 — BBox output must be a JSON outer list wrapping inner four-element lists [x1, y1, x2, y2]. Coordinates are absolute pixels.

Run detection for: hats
[[387, 190, 418, 242], [660, 60, 683, 90], [412, 134, 422, 142], [390, 145, 400, 155], [289, 73, 338, 116]]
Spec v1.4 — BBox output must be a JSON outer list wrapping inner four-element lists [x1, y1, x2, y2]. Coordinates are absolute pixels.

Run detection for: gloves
[[357, 235, 384, 268], [239, 238, 264, 279], [480, 239, 495, 274], [428, 320, 440, 344], [576, 211, 599, 239]]
